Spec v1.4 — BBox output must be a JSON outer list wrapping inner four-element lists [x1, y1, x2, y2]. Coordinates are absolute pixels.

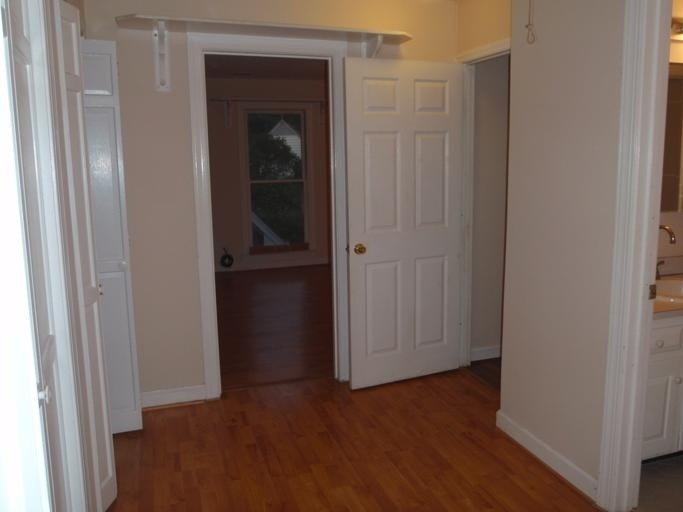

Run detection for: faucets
[[659, 224, 677, 245]]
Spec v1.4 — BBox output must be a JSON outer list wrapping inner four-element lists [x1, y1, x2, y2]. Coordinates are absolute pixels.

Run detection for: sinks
[[656, 280, 683, 304]]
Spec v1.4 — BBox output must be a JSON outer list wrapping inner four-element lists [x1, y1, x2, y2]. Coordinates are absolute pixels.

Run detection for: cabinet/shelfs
[[640, 312, 683, 465]]
